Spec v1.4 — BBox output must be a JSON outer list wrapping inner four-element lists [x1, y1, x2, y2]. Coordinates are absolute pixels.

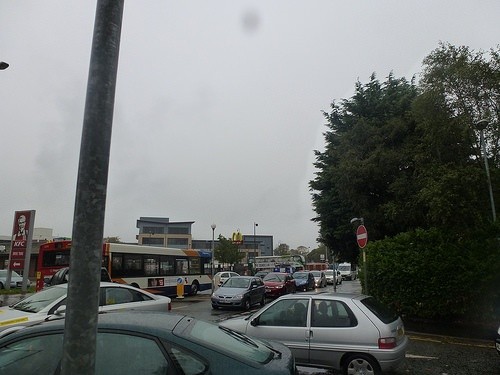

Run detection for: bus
[[252, 255, 306, 272], [35, 240, 213, 297], [273, 262, 304, 274]]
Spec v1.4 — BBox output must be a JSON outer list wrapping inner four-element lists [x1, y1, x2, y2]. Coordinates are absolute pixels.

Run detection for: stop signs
[[356, 225, 368, 249]]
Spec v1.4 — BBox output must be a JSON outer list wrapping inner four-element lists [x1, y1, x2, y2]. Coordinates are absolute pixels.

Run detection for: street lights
[[253, 220, 258, 270], [476, 119, 497, 223], [210, 223, 216, 278], [0, 244, 6, 270]]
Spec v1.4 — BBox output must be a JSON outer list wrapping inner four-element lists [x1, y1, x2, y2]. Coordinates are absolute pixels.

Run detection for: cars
[[323, 269, 342, 286], [309, 270, 327, 288], [263, 272, 296, 298], [292, 272, 315, 291], [0, 282, 171, 339], [210, 275, 266, 310], [254, 270, 274, 277], [211, 272, 241, 291], [216, 291, 409, 375], [0, 270, 29, 293], [0, 309, 297, 375]]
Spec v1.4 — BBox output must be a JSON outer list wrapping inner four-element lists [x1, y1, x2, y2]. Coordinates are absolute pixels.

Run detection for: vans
[[337, 262, 358, 281]]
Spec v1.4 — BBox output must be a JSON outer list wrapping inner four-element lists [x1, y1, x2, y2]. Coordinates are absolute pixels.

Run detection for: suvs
[[43, 265, 112, 285]]
